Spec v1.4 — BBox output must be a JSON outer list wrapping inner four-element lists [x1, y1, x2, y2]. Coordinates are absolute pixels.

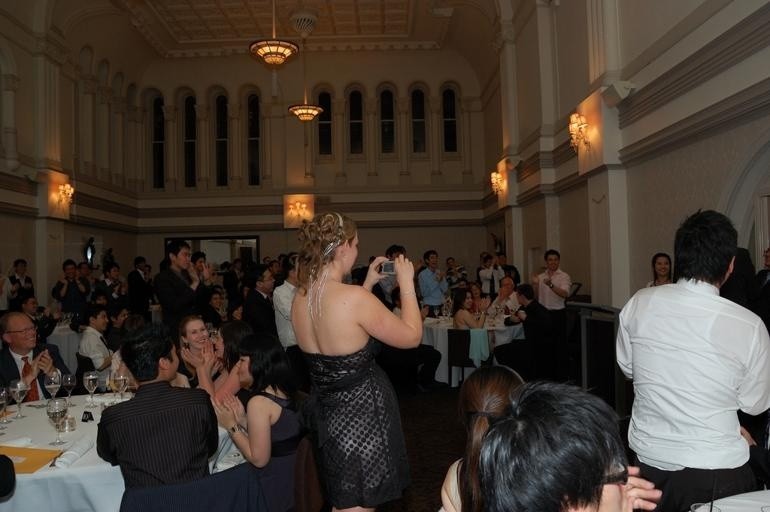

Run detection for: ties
[[21, 357, 39, 402]]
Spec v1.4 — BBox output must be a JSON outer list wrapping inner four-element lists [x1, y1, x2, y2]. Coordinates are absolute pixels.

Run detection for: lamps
[[56, 182, 77, 208], [287, 59, 326, 123], [565, 109, 593, 154], [246, 0, 301, 67], [489, 169, 507, 195], [288, 200, 310, 219]]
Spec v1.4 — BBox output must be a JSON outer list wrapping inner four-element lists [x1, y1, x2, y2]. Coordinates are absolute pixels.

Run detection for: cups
[[691, 503, 722, 512], [434, 303, 519, 326]]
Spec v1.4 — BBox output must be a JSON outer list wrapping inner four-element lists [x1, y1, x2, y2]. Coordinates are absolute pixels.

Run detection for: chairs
[[447, 328, 496, 393], [288, 435, 328, 512], [69, 350, 102, 397]]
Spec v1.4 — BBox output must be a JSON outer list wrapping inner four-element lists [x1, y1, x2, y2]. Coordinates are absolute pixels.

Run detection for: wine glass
[[45, 398, 69, 446], [36, 310, 77, 327], [0, 367, 132, 436]]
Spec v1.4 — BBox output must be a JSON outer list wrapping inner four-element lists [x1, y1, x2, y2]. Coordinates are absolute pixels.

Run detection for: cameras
[[378, 260, 397, 275]]
